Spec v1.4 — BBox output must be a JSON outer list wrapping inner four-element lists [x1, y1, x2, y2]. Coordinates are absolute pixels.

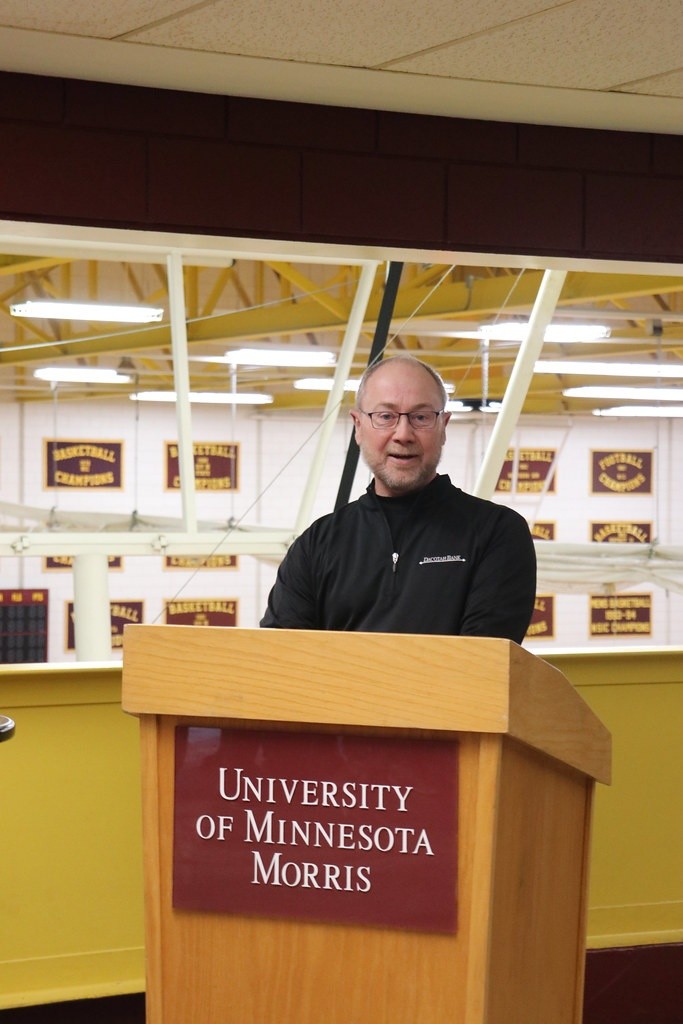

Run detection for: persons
[[260, 354, 537, 647]]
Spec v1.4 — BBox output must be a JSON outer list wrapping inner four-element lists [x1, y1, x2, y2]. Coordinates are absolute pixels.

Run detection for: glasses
[[358, 407, 445, 431]]
